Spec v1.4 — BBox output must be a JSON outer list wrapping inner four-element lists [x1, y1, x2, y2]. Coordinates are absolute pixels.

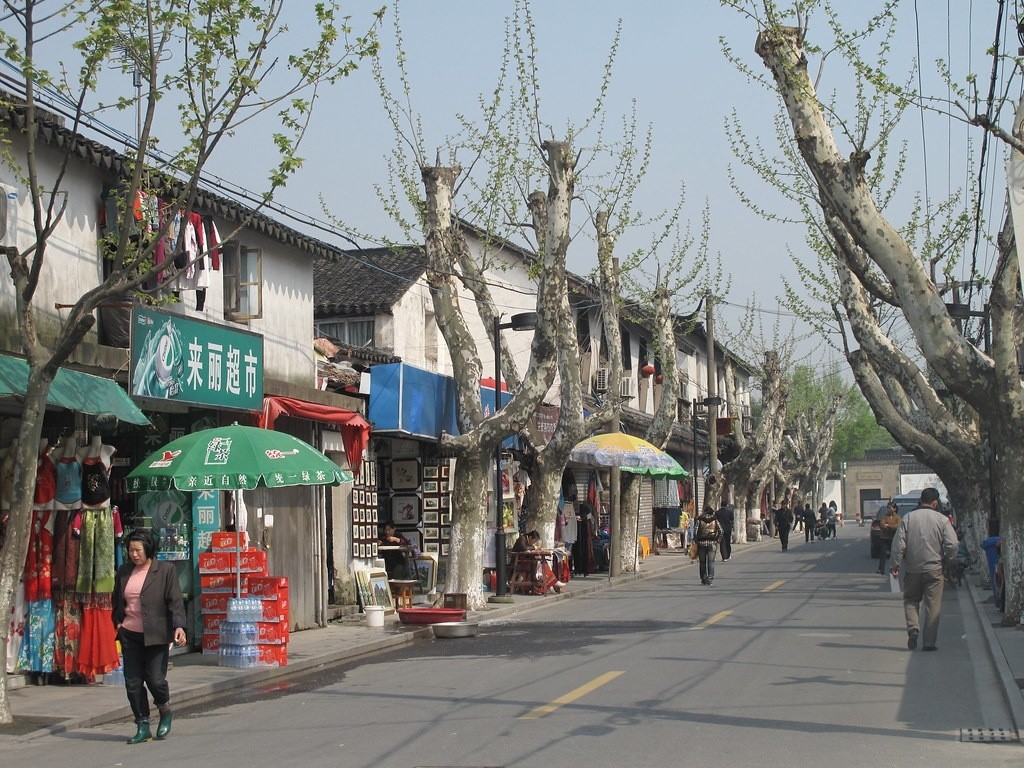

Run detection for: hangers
[[117, 176, 132, 182]]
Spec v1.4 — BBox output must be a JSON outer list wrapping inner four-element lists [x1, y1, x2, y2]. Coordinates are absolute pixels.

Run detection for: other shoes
[[729, 553, 731, 559], [722, 559, 727, 562], [907, 629, 918, 649], [923, 646, 937, 651], [876, 571, 884, 575]]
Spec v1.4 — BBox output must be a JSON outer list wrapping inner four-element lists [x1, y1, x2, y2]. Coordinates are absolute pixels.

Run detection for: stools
[[394, 583, 413, 608]]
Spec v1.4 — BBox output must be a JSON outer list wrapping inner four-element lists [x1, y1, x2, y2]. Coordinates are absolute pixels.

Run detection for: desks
[[377, 545, 424, 596], [653, 530, 687, 556], [510, 552, 561, 597]]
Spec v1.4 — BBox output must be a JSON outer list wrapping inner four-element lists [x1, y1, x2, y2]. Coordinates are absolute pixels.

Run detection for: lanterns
[[641, 364, 663, 390]]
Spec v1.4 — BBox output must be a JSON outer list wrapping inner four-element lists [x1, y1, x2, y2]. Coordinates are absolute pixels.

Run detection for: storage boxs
[[199, 531, 290, 666]]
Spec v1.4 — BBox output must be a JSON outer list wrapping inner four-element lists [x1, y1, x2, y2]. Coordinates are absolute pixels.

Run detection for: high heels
[[549, 581, 566, 593]]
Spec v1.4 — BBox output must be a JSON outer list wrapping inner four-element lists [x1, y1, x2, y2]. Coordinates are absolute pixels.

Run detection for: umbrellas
[[571, 433, 692, 583], [130, 423, 354, 600]]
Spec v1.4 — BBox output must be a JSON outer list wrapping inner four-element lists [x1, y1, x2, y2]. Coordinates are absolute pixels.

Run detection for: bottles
[[135, 334, 174, 398], [101, 653, 126, 686], [121, 511, 189, 552], [217, 593, 264, 669]]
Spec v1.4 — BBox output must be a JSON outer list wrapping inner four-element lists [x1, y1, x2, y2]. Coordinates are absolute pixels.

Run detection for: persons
[[890, 487, 959, 652], [694, 506, 723, 587], [715, 502, 735, 562], [802, 503, 817, 544], [790, 500, 804, 534], [875, 501, 901, 575], [771, 500, 780, 537], [375, 521, 411, 596], [818, 501, 837, 540], [109, 533, 188, 744], [510, 531, 566, 596], [0, 435, 116, 510], [775, 500, 794, 553]]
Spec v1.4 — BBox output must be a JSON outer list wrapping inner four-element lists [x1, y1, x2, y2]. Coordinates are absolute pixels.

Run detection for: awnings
[[0, 354, 154, 426], [261, 398, 370, 476]]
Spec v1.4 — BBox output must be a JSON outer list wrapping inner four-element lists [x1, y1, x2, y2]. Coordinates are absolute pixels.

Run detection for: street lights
[[947, 301, 1000, 537], [492, 312, 541, 597], [692, 397, 722, 539]]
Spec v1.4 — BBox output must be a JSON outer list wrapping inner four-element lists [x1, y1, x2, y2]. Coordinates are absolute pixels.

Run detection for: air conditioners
[[596, 367, 609, 391], [744, 419, 752, 432], [620, 377, 637, 398]]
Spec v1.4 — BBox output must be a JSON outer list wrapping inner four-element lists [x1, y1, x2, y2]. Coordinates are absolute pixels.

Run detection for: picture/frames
[[391, 528, 450, 595], [390, 459, 421, 491], [441, 496, 449, 508], [424, 498, 439, 510], [441, 467, 449, 478], [423, 480, 438, 494], [423, 512, 438, 523], [351, 457, 389, 558], [441, 513, 450, 525], [390, 493, 422, 527], [423, 464, 439, 479], [440, 481, 449, 493]]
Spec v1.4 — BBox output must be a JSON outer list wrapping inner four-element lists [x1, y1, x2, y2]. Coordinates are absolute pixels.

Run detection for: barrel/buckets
[[365, 605, 384, 627]]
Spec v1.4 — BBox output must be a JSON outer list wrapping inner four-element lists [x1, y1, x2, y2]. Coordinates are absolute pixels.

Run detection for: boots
[[156, 701, 173, 737], [127, 717, 152, 743]]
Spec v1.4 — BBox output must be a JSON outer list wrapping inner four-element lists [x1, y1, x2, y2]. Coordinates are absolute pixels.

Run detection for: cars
[[871, 503, 915, 560]]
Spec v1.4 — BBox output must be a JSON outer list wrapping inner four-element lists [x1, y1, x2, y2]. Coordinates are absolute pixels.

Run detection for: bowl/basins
[[432, 621, 478, 637], [398, 608, 465, 625]]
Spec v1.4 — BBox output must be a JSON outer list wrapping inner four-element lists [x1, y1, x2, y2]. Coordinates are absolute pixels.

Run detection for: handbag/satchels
[[688, 539, 698, 560]]
